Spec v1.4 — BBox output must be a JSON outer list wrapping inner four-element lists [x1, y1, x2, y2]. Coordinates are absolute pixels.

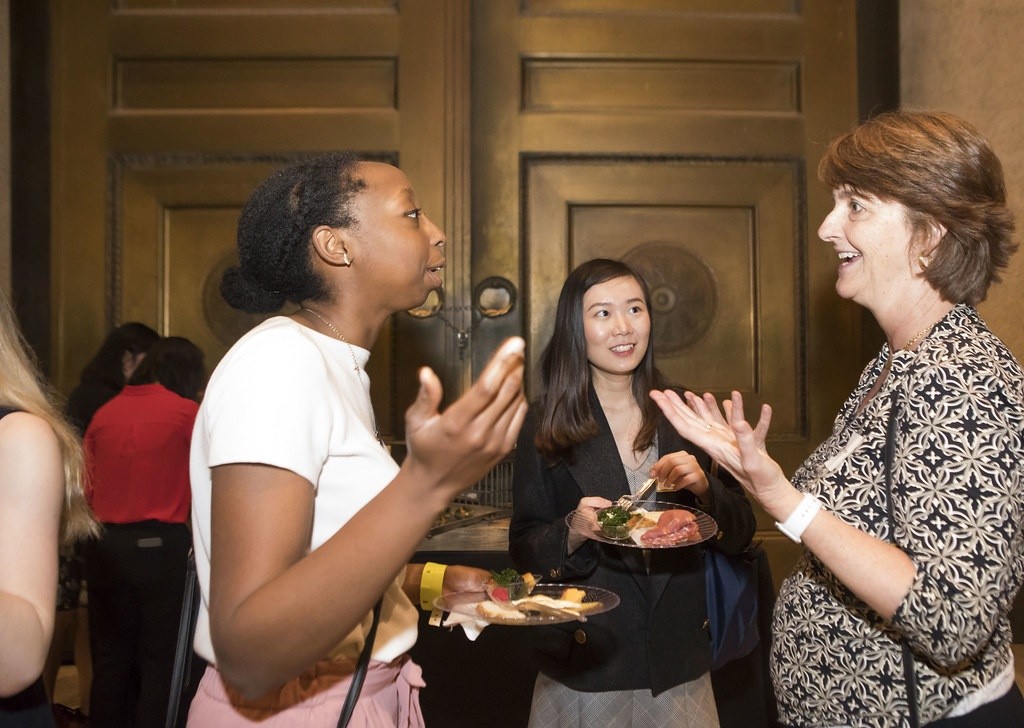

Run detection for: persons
[[66, 322, 159, 433], [86, 337, 203, 728], [507, 258, 757, 728], [649, 111, 1023, 728], [186, 151, 529, 727], [0, 289, 101, 728]]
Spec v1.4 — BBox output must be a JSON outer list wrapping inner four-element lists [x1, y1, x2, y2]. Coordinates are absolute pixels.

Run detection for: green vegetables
[[597, 505, 633, 540], [488, 567, 526, 600]]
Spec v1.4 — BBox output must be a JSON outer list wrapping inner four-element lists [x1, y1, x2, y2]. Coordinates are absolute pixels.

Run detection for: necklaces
[[306, 309, 385, 446], [903, 322, 939, 349]]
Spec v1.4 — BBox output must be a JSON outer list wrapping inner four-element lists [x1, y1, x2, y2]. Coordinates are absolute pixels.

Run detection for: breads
[[475, 593, 604, 620]]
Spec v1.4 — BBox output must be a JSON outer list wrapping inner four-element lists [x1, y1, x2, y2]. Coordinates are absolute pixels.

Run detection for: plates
[[565, 500, 718, 548], [432, 583, 620, 625]]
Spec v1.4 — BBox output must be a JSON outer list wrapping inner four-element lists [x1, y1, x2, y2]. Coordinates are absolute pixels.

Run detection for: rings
[[707, 425, 712, 429]]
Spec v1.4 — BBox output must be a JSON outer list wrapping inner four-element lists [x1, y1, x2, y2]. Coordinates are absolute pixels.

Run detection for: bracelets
[[420, 562, 446, 627]]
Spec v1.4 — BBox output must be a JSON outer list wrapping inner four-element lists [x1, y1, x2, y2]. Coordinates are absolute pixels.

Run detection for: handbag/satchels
[[703, 459, 775, 671]]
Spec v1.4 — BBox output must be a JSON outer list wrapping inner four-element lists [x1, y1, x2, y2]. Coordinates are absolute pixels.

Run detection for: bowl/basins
[[482, 575, 542, 600], [593, 503, 646, 542]]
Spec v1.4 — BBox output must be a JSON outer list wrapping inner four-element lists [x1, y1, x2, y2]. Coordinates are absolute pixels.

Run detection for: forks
[[489, 591, 587, 623], [615, 477, 656, 510]]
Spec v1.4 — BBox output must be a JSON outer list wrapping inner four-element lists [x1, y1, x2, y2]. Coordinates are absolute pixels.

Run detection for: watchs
[[775, 493, 822, 544]]
